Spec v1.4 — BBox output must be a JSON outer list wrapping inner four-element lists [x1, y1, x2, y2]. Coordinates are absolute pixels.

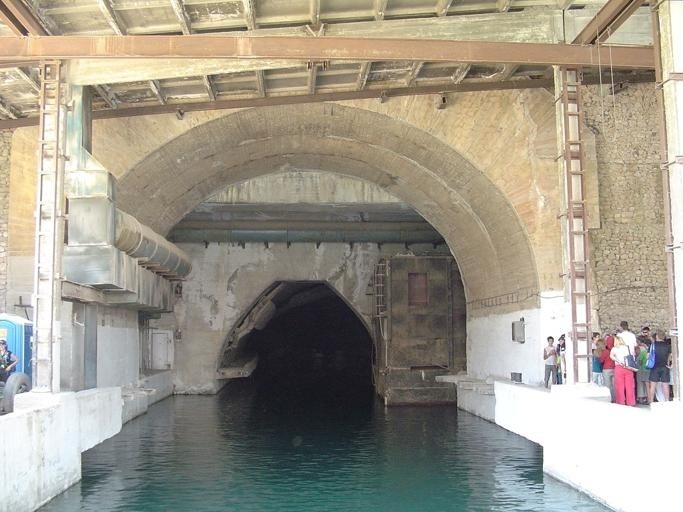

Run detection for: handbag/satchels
[[645, 342, 656, 369], [623, 345, 640, 372]]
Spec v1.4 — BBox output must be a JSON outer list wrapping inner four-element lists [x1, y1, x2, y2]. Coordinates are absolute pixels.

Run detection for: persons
[[544, 321, 674, 407], [0, 340, 19, 383]]
